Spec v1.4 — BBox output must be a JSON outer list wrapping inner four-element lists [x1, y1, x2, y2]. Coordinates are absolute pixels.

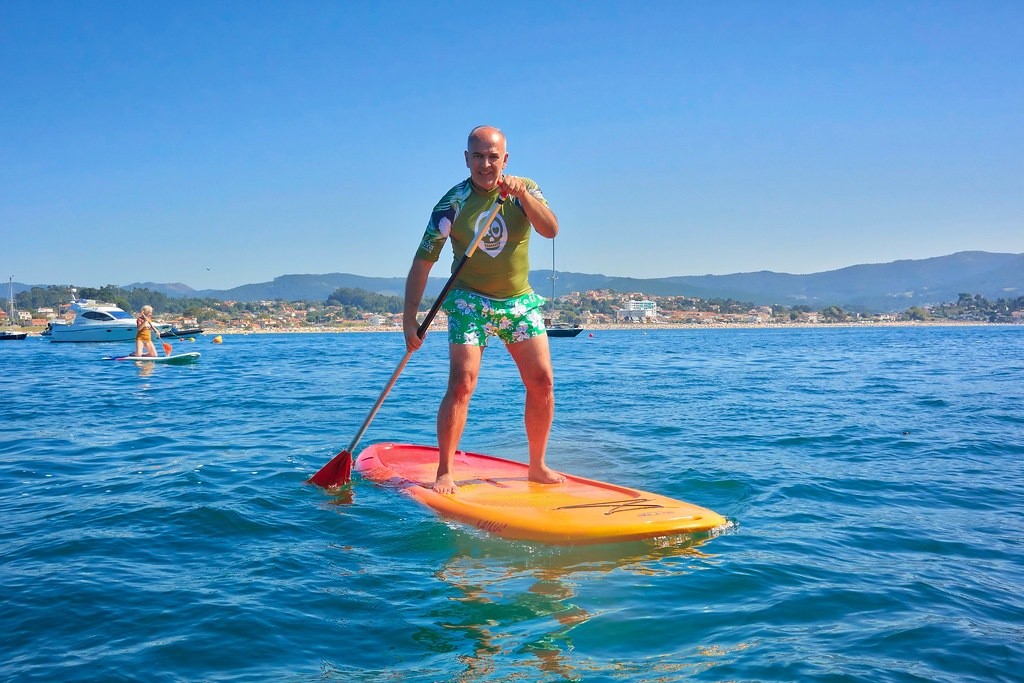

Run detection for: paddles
[[140, 310, 172, 355], [307, 178, 510, 488]]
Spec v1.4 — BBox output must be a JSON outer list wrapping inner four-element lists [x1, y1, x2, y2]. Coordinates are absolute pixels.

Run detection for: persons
[[128, 304, 161, 357], [402, 125, 569, 496]]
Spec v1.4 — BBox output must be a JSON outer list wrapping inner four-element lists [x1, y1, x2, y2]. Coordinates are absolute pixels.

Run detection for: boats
[[49, 300, 172, 343], [102, 352, 202, 365], [356, 441, 731, 549], [160, 329, 204, 338]]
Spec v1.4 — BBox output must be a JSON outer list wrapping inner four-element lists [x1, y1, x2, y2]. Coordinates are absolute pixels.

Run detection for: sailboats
[[0, 277, 28, 340], [542, 237, 583, 337]]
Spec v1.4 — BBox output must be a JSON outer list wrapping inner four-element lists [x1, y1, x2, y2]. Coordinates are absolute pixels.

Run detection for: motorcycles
[[41, 330, 51, 335]]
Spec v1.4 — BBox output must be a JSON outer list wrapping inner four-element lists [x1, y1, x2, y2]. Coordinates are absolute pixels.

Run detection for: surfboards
[[101, 352, 201, 362], [353, 441, 727, 546]]
